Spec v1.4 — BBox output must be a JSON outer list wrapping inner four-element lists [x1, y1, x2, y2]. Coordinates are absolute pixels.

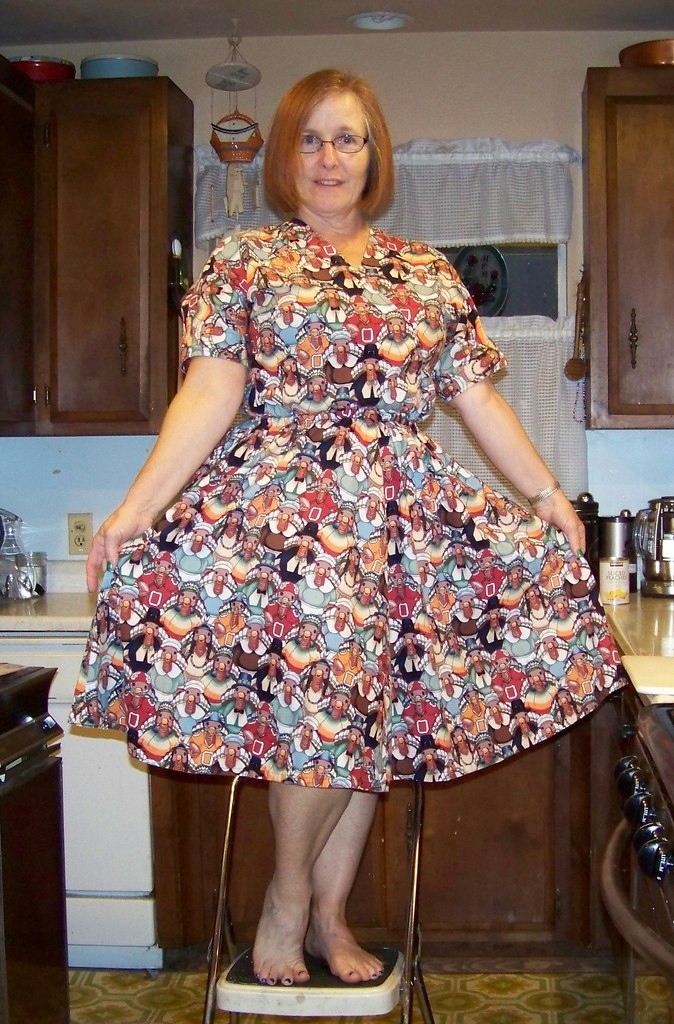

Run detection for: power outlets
[[68, 512, 93, 555]]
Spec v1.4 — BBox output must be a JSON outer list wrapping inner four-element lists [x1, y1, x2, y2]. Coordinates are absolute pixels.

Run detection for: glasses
[[297, 134, 369, 154]]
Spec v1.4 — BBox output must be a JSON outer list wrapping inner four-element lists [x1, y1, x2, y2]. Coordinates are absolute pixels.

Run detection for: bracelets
[[528, 481, 561, 507]]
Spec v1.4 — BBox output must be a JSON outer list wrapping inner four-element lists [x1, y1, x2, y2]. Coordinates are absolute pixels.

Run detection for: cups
[[28, 551, 46, 597], [599, 558, 629, 604]]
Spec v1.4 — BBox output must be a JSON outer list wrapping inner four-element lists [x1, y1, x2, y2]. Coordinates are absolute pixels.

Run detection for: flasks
[[566, 492, 600, 592]]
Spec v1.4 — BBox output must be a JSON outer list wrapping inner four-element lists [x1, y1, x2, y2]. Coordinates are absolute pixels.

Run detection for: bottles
[[0, 508, 35, 599]]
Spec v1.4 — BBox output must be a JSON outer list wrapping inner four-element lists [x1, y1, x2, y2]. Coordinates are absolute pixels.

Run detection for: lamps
[[349, 12, 413, 31]]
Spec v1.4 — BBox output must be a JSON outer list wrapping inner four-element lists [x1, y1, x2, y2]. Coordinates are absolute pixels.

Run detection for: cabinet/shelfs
[[582, 64, 673, 430], [0, 55, 38, 437], [148, 694, 614, 974], [35, 77, 193, 439]]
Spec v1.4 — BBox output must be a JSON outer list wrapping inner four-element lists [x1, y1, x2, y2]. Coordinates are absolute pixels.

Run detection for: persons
[[66, 67, 624, 985]]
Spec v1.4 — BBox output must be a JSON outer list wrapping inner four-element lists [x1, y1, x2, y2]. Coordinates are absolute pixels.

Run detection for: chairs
[[197, 752, 439, 1024]]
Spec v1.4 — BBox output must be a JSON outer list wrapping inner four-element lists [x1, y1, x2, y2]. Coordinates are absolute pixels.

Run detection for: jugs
[[632, 495, 673, 582]]
